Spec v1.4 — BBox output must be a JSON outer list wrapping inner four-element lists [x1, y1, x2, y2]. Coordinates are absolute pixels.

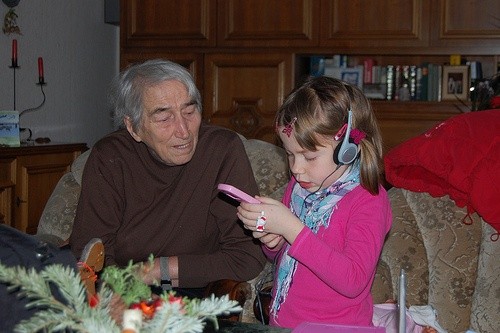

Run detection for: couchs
[[38, 130, 291, 326], [359, 187, 499, 333]]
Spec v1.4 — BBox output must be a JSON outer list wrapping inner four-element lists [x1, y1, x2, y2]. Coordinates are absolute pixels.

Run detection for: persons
[[238, 75, 392, 333], [70, 58, 269, 300]]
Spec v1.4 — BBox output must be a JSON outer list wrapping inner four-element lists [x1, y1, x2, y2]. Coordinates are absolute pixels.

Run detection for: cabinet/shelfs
[[0, 142, 88, 237], [120, 1, 500, 145]]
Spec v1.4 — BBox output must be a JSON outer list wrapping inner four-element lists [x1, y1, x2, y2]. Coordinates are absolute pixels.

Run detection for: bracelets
[[160, 257, 173, 294]]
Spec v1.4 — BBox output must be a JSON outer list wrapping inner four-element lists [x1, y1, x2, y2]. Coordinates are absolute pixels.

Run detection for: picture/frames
[[441, 65, 469, 102]]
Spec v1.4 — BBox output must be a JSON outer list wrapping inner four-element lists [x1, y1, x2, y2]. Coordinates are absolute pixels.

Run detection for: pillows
[[385, 110, 500, 235]]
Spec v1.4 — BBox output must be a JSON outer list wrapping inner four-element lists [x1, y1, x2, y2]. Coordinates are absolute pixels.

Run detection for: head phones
[[333, 85, 358, 166]]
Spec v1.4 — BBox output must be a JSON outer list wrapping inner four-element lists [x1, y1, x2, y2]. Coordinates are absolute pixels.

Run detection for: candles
[[12, 39, 18, 66], [38, 56, 45, 83]]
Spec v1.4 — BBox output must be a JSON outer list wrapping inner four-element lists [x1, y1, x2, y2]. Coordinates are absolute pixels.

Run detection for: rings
[[255, 211, 266, 232]]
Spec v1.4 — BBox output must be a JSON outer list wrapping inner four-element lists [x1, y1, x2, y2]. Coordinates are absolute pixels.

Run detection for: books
[[310, 55, 441, 101]]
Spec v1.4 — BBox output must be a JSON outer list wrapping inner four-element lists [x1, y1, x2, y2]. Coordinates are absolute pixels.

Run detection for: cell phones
[[217, 184, 262, 205]]
